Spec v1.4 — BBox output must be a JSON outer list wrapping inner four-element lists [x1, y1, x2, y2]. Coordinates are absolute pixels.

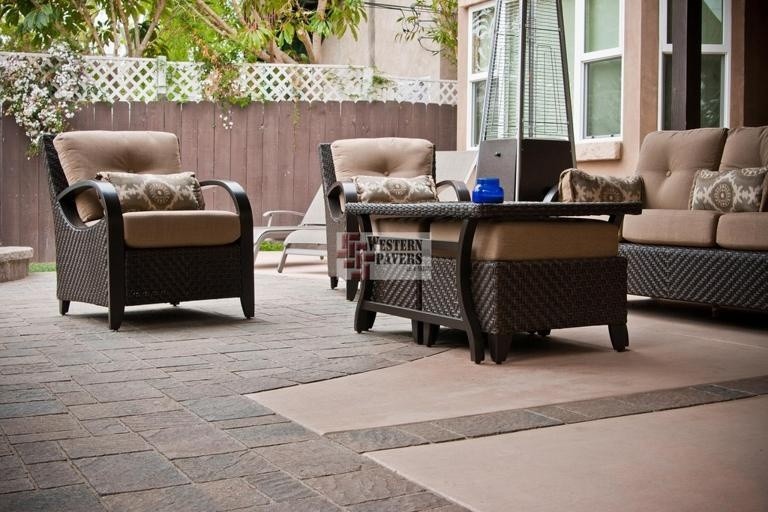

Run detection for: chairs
[[319, 140, 471, 301], [36, 133, 260, 330]]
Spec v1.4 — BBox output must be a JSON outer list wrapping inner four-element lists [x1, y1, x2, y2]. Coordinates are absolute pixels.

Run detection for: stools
[[422, 219, 629, 365], [363, 216, 537, 344]]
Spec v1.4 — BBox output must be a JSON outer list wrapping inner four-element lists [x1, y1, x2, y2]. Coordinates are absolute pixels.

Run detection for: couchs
[[542, 169, 767, 312]]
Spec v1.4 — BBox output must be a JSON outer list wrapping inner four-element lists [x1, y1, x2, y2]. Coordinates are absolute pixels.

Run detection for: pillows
[[687, 166, 767, 213], [559, 167, 644, 202], [95, 170, 206, 215], [330, 136, 434, 213], [718, 126, 768, 171], [632, 127, 727, 210], [352, 176, 439, 203], [51, 130, 182, 222]]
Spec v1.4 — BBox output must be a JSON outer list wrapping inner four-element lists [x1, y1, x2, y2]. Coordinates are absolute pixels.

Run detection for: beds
[[344, 201, 642, 364]]
